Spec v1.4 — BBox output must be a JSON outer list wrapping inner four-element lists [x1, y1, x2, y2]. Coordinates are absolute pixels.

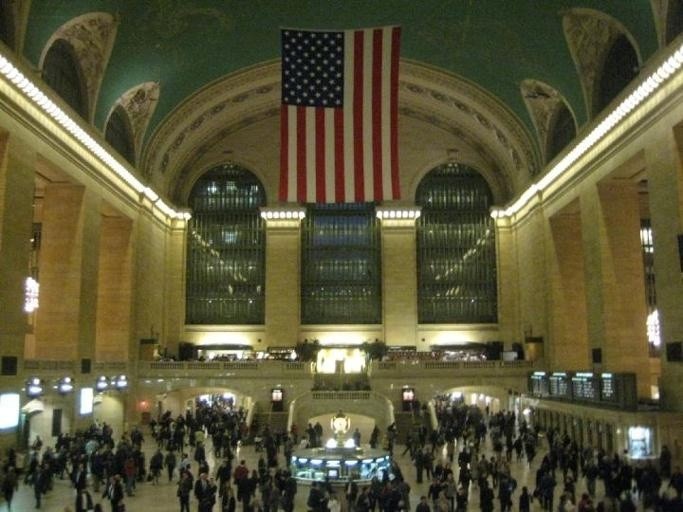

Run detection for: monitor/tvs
[[80, 386, 93, 415], [271, 388, 283, 402], [0, 392, 22, 431], [627, 425, 650, 457], [401, 387, 415, 401]]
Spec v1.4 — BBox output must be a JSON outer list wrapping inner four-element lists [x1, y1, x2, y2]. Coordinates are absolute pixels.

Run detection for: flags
[[275, 22, 406, 206]]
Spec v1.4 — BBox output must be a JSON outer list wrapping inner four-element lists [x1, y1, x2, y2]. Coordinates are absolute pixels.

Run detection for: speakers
[[80, 357, 92, 373], [0, 356, 18, 376], [591, 347, 602, 364], [666, 342, 683, 363]]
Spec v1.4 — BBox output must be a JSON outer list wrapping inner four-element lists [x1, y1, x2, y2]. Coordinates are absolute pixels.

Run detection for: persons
[[385, 349, 491, 361], [156, 350, 301, 362]]
